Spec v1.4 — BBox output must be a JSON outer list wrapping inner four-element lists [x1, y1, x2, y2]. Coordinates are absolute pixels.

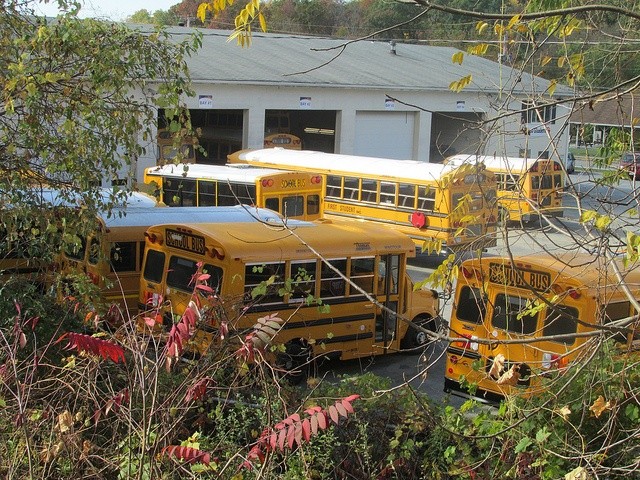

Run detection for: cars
[[568, 153, 576, 173]]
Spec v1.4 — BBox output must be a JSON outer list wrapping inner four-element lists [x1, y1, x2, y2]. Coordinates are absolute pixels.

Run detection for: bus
[[443, 154, 564, 223], [0, 183, 168, 289], [444, 253, 640, 410], [136, 222, 439, 385], [143, 163, 328, 222], [57, 204, 285, 328], [227, 147, 498, 258], [157, 129, 196, 165], [197, 128, 302, 165]]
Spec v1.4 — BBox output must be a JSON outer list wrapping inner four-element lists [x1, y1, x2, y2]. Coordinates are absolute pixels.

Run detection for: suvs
[[619, 153, 640, 179]]
